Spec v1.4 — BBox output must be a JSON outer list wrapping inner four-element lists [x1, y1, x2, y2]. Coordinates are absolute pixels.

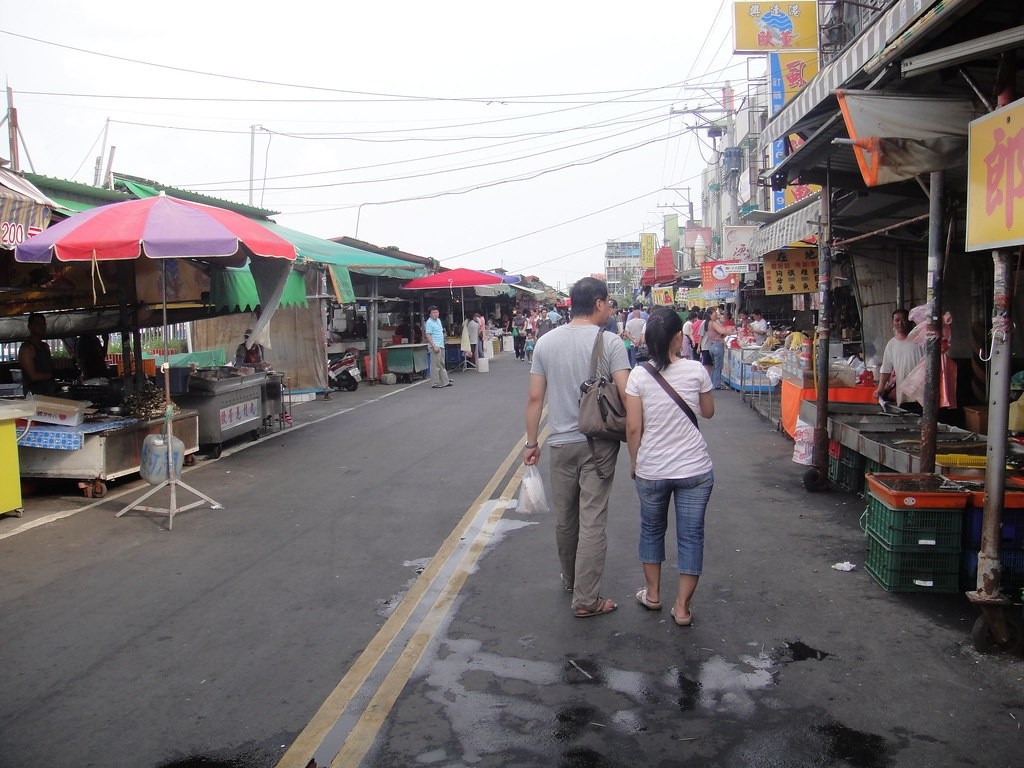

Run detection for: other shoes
[[444, 383, 453, 387], [522, 357, 524, 361], [282, 413, 292, 424], [712, 384, 729, 390], [432, 385, 442, 388], [528, 361, 532, 364]]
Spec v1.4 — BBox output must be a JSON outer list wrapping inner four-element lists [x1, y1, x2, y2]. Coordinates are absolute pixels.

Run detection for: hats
[[609, 299, 618, 308]]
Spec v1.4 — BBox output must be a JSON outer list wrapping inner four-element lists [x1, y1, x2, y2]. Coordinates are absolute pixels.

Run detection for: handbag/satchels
[[515, 465, 550, 515], [513, 327, 518, 336], [578, 327, 627, 443], [635, 342, 649, 362]]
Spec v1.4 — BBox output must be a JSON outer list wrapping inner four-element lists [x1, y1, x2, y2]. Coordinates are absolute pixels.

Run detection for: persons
[[425, 305, 453, 389], [873, 309, 926, 414], [235, 330, 292, 423], [522, 277, 632, 617], [463, 306, 571, 369], [75, 331, 110, 381], [784, 332, 810, 351], [626, 306, 716, 626], [19, 312, 63, 398], [354, 316, 367, 340], [599, 298, 766, 390]]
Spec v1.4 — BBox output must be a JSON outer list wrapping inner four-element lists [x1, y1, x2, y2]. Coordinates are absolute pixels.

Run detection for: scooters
[[326, 350, 361, 392]]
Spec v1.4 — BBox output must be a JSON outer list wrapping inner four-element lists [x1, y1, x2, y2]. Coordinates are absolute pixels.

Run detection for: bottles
[[800, 340, 813, 370], [139, 434, 185, 486]]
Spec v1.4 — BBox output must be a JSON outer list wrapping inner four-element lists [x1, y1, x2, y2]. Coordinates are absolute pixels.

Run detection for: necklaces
[[572, 319, 589, 321]]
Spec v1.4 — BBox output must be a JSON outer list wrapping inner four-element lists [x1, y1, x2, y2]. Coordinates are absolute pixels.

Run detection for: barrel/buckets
[[478, 357, 489, 373]]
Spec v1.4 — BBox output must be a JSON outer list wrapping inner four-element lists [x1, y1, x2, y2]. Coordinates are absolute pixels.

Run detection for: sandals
[[576, 596, 617, 616], [560, 570, 573, 592]]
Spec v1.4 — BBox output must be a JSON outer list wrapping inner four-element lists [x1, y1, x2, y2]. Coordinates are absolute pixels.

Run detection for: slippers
[[636, 589, 662, 610], [671, 606, 692, 625]]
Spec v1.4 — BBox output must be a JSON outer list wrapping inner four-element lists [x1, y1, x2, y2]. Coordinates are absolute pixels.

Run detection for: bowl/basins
[[9, 368, 23, 383], [102, 405, 130, 416]]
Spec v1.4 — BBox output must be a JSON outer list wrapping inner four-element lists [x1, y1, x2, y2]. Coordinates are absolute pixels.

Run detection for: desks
[[741, 360, 798, 430], [780, 379, 879, 441], [729, 346, 777, 394], [720, 347, 730, 388], [379, 343, 429, 384], [445, 343, 477, 371], [0, 398, 36, 518]]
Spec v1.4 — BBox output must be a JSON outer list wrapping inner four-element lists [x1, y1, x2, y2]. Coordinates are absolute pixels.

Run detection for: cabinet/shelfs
[[829, 341, 862, 366]]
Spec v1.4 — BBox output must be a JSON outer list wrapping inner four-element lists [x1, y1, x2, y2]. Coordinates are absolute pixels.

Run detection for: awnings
[[638, 246, 677, 286]]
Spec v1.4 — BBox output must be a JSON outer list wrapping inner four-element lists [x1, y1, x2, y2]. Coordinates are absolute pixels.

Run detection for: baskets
[[936, 454, 1013, 469]]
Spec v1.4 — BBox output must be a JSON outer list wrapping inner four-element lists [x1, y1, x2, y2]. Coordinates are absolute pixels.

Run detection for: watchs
[[525, 441, 538, 450]]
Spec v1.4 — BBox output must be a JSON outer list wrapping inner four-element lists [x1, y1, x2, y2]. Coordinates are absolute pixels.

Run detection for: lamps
[[901, 25, 1024, 81]]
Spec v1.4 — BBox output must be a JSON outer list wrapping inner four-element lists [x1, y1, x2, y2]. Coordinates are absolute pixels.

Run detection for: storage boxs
[[935, 453, 1014, 469], [378, 330, 396, 339], [803, 364, 856, 388], [401, 338, 409, 344], [840, 446, 868, 470], [382, 337, 393, 341], [862, 531, 961, 595], [962, 507, 1024, 548], [798, 399, 921, 429], [69, 384, 113, 402], [857, 429, 988, 464], [865, 479, 870, 494], [384, 326, 398, 331], [328, 341, 366, 353], [838, 461, 865, 494], [382, 341, 393, 347], [863, 493, 963, 553], [281, 392, 316, 403], [865, 472, 974, 509], [10, 369, 23, 383], [863, 490, 872, 505], [827, 439, 844, 460], [827, 456, 840, 483], [16, 418, 37, 426], [827, 415, 972, 452], [392, 335, 403, 344], [864, 458, 892, 472], [909, 442, 1024, 476], [21, 395, 98, 426], [945, 473, 1024, 509], [881, 433, 988, 473], [0, 384, 24, 395], [963, 546, 1024, 580], [964, 405, 988, 435]]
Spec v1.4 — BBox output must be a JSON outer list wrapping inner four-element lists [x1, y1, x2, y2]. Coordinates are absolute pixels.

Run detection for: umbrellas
[[400, 266, 520, 362], [14, 190, 298, 480]]
